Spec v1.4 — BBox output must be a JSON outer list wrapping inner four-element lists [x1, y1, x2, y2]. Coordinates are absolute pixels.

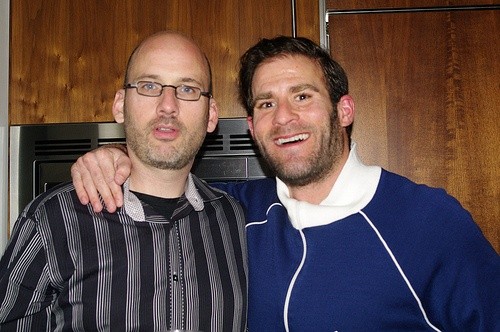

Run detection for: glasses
[[123, 81, 211, 101]]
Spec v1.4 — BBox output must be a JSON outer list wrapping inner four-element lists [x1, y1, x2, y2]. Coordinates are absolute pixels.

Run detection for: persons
[[70, 35, 500, 331], [0, 31, 249, 332]]
